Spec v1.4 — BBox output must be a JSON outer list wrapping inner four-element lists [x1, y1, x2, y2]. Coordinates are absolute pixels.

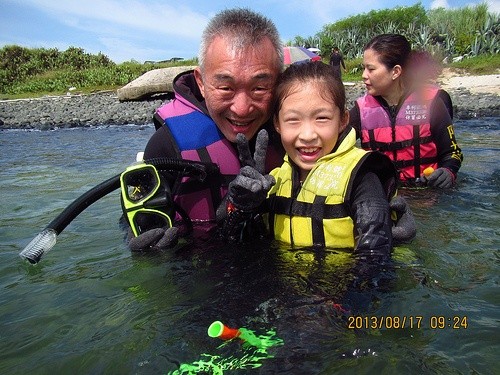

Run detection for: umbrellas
[[281, 45, 321, 65], [306, 47, 321, 53]]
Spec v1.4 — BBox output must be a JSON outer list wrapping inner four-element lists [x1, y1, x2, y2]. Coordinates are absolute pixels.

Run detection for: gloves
[[389, 196, 416, 238], [125, 227, 181, 250], [228, 129, 275, 210], [427, 168, 453, 189]]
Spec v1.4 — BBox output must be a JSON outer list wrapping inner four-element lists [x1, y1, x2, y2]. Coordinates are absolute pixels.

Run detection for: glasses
[[120, 164, 172, 237]]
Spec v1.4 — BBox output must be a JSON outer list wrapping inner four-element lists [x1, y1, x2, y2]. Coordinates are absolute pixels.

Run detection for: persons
[[328, 46, 346, 81], [217, 58, 391, 256], [345, 32, 464, 194], [117, 7, 418, 253]]
